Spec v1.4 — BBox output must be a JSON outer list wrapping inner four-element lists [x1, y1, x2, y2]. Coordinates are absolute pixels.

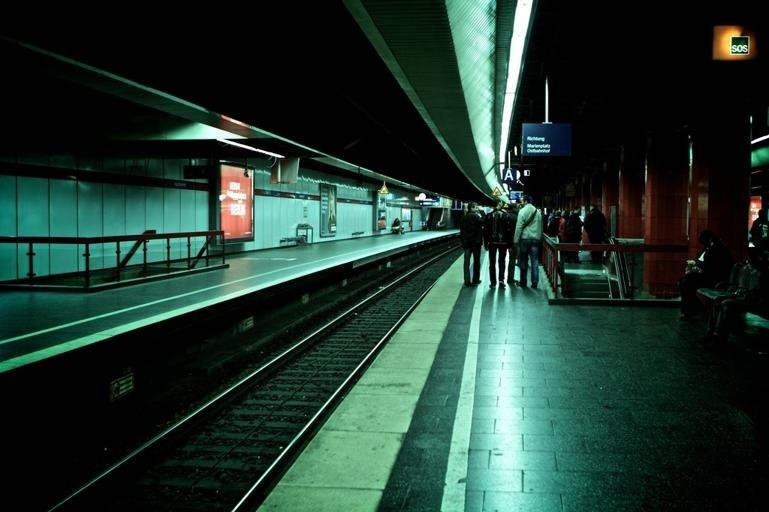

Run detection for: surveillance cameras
[[244, 172, 249, 178]]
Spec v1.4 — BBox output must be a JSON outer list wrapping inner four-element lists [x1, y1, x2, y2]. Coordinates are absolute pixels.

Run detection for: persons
[[391, 217, 403, 235], [750, 208, 769, 248], [676, 227, 733, 322], [458, 194, 608, 291], [694, 237, 768, 355]]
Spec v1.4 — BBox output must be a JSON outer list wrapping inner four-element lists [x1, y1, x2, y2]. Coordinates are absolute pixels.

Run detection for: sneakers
[[464, 278, 538, 289]]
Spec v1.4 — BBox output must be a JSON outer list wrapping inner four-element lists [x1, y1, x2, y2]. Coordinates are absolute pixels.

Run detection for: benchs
[[696, 262, 769, 360], [280, 235, 308, 248]]
